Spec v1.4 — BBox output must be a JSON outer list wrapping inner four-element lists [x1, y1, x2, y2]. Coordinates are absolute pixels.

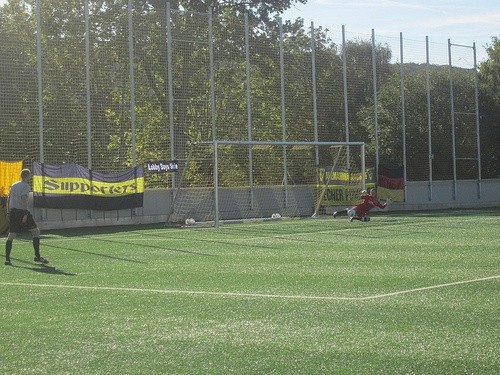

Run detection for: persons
[[4, 169, 49, 265], [333, 187, 392, 222]]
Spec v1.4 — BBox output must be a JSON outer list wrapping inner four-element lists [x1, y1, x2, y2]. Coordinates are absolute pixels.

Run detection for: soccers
[[347, 210, 355, 216]]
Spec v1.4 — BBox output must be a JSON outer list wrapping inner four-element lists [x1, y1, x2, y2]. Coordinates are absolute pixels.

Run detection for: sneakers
[[5, 260, 12, 265], [34, 256, 49, 265]]
